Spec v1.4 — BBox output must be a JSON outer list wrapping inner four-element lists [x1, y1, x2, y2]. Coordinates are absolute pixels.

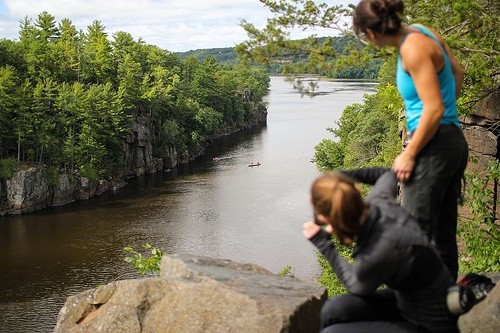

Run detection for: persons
[[303, 166, 462, 333], [353, 1, 469, 283]]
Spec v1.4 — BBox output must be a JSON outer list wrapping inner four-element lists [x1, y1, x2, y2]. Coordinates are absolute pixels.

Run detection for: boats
[[248, 163, 261, 167], [210, 157, 219, 161]]
